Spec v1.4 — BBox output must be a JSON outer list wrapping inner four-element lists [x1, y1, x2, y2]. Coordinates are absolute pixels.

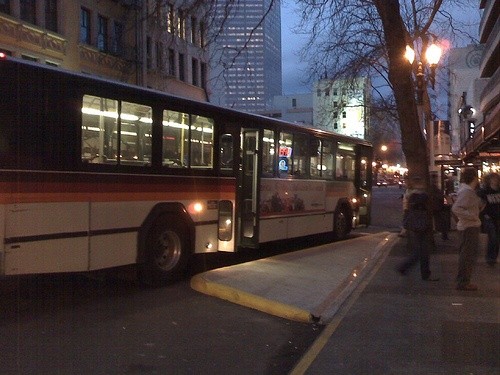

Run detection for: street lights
[[403, 43, 443, 140]]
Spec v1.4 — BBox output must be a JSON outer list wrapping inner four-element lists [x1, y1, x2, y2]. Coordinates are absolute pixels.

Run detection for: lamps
[[405, 40, 442, 105]]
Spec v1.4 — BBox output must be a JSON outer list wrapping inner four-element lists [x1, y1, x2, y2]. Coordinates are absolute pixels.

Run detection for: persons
[[479, 173, 500, 269], [395, 174, 488, 282], [451, 168, 482, 290]]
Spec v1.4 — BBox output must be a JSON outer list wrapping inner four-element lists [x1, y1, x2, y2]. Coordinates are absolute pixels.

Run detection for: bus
[[0, 51, 375, 290]]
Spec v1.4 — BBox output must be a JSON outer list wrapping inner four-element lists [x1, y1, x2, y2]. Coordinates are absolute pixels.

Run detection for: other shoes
[[456, 282, 479, 290]]
